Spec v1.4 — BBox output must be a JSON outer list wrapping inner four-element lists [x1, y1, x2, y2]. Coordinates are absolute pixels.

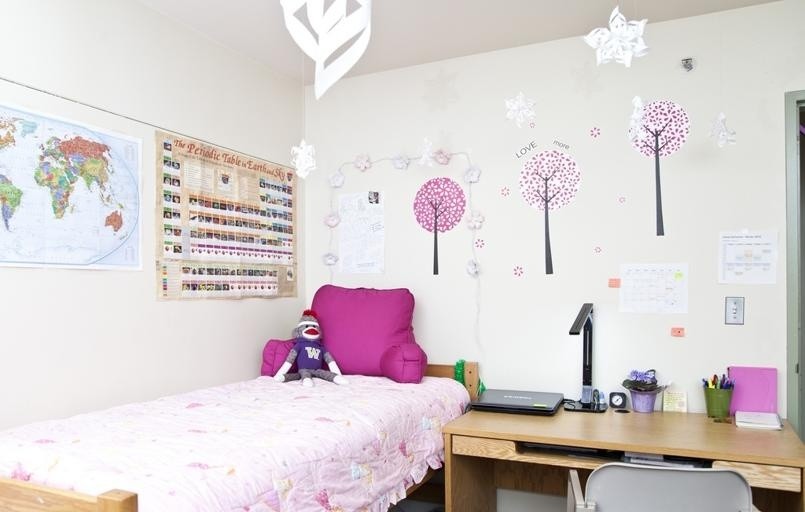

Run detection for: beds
[[0, 362, 478, 512]]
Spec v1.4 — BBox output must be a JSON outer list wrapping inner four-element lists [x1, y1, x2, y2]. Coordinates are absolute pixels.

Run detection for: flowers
[[622, 370, 662, 393]]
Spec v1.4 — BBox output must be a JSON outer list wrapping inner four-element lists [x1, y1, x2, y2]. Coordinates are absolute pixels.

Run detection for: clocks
[[609, 392, 626, 408]]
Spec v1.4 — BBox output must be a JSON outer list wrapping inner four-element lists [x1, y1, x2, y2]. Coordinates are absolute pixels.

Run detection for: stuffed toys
[[273, 310, 347, 389]]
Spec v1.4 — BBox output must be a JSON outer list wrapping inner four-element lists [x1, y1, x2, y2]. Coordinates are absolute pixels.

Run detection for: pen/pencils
[[702, 373, 735, 389]]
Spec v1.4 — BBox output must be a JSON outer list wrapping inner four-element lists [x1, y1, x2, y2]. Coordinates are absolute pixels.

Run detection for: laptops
[[471, 389, 564, 416]]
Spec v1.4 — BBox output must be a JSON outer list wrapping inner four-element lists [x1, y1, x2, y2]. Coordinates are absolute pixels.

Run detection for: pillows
[[261, 285, 426, 383]]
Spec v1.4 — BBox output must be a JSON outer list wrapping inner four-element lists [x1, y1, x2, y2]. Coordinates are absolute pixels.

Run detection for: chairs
[[566, 462, 760, 512]]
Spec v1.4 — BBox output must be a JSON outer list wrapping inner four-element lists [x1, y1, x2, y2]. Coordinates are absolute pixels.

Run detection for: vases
[[629, 389, 656, 413]]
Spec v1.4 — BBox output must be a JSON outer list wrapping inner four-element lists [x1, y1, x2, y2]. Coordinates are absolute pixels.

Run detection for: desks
[[442, 403, 805, 512]]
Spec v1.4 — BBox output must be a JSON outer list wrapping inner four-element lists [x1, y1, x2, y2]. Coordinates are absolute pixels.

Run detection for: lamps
[[563, 304, 608, 413]]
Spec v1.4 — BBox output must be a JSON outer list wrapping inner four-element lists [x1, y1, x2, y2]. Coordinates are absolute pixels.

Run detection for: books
[[734, 409, 784, 432], [517, 443, 693, 469]]
